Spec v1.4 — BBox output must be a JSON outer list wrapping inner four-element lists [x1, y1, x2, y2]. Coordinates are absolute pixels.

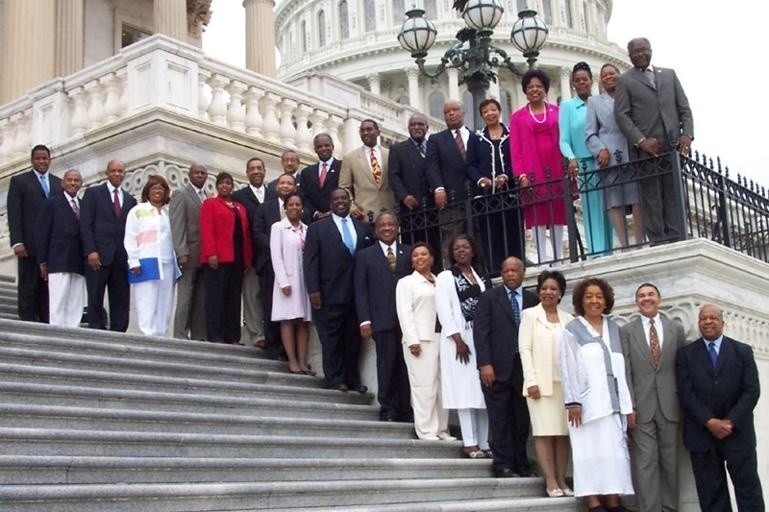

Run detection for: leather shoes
[[461, 444, 488, 459], [253, 337, 268, 349], [401, 412, 415, 422], [438, 433, 458, 441], [328, 379, 350, 391], [509, 460, 537, 477], [493, 460, 514, 478], [416, 432, 440, 440], [380, 410, 400, 422], [346, 380, 368, 393]]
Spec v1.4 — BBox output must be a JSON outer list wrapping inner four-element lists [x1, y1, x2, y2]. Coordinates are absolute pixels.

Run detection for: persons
[[5, 144, 254, 346], [233, 37, 768, 512]]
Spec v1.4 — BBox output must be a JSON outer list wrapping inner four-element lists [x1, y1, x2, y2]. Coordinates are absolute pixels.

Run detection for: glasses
[[630, 46, 651, 56]]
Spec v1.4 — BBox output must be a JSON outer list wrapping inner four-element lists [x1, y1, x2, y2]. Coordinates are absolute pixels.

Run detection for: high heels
[[544, 485, 564, 498], [304, 369, 317, 376], [287, 363, 305, 375], [561, 486, 576, 497]]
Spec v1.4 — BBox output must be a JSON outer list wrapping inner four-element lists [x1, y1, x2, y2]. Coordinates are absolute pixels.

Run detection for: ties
[[648, 317, 662, 365], [70, 197, 81, 220], [369, 147, 382, 189], [643, 68, 657, 89], [198, 190, 206, 204], [707, 340, 719, 370], [417, 143, 426, 159], [113, 187, 124, 217], [341, 218, 355, 257], [509, 290, 521, 332], [454, 129, 467, 166], [40, 174, 50, 198], [319, 161, 328, 188], [387, 247, 397, 272]]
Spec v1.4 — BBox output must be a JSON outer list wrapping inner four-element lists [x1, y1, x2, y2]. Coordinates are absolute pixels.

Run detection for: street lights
[[397, 0, 548, 128]]
